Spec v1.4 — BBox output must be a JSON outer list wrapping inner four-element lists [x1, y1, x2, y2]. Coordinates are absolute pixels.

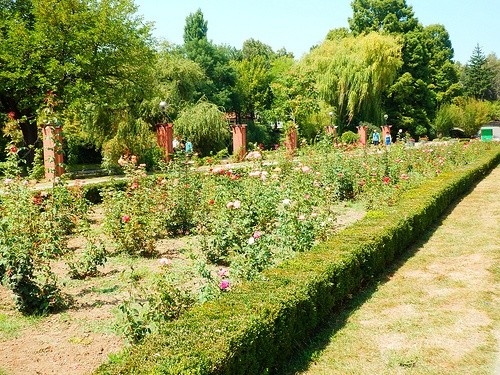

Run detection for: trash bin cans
[[480, 128, 493, 142]]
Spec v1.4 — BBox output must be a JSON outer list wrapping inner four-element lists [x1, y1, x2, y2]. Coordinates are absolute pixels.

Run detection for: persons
[[185, 138, 193, 152], [372, 129, 380, 149], [384, 131, 392, 152], [172, 135, 180, 149]]
